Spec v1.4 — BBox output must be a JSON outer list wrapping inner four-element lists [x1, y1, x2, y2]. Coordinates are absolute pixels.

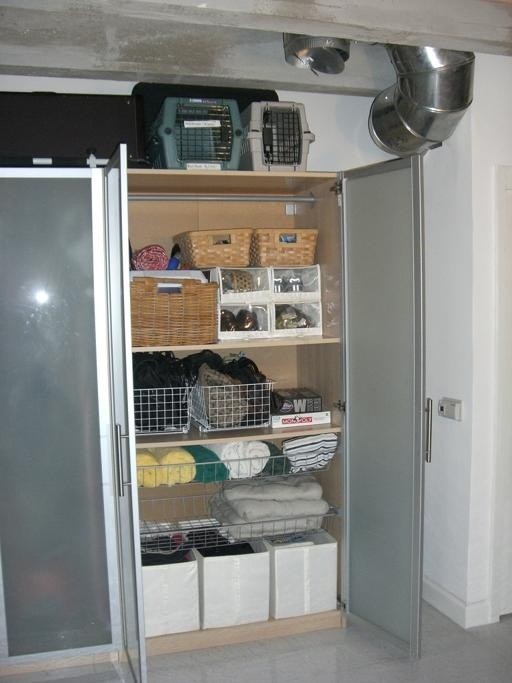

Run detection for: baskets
[[131, 276, 221, 346], [133, 385, 194, 436], [172, 227, 252, 269], [250, 227, 320, 267], [191, 376, 278, 432]]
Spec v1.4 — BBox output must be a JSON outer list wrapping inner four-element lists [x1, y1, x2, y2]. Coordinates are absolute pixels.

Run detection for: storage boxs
[[139, 516, 341, 641]]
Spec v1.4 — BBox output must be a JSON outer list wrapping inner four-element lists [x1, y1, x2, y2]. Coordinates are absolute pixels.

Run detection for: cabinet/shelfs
[[105, 142, 426, 681], [0, 168, 120, 678]]
